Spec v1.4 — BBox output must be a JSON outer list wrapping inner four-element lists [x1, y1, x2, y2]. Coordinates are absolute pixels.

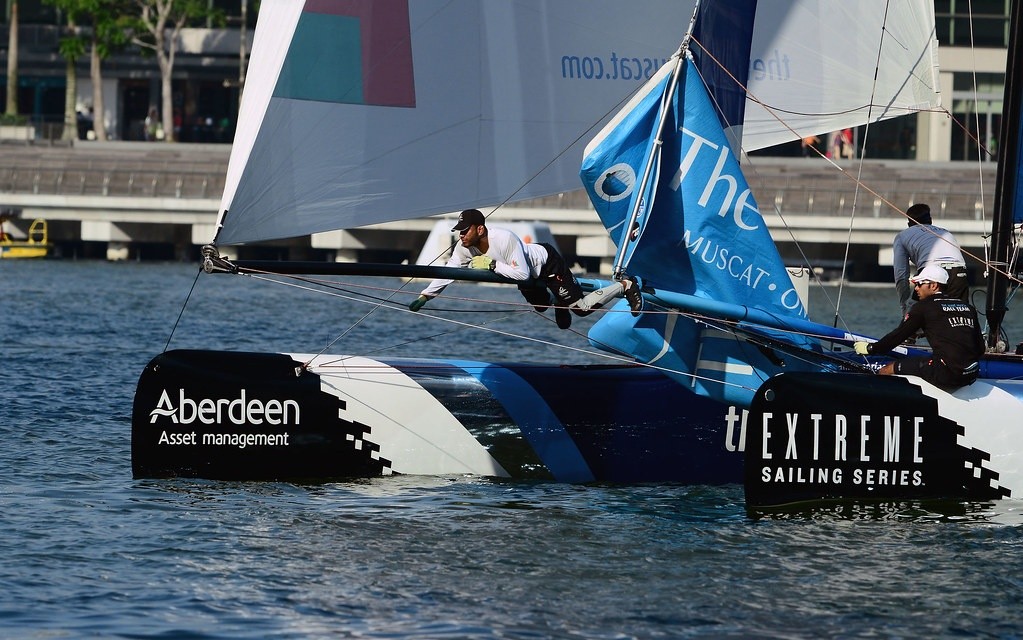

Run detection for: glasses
[[917, 280, 934, 288]]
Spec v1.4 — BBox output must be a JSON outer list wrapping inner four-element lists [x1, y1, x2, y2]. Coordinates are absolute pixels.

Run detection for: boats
[[0, 215, 56, 259], [394, 215, 590, 287]]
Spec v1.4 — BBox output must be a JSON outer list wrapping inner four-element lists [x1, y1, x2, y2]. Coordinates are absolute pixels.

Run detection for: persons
[[74, 106, 165, 143], [408, 209, 643, 328], [892, 204, 970, 344], [854, 266, 984, 394]]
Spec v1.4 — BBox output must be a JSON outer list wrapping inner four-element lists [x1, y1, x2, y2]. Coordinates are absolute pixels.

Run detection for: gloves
[[901, 305, 906, 317], [409, 294, 427, 312], [471, 256, 495, 270], [853, 342, 871, 355]]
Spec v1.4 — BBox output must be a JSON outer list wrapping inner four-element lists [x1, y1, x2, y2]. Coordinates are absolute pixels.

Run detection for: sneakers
[[623, 275, 644, 317], [554, 300, 572, 329]]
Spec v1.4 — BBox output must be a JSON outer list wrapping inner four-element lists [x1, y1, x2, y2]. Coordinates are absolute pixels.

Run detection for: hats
[[912, 265, 950, 285], [451, 210, 484, 232]]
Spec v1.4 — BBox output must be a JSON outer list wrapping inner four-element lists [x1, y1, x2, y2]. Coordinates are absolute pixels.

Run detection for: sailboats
[[125, 2, 1023, 507]]
